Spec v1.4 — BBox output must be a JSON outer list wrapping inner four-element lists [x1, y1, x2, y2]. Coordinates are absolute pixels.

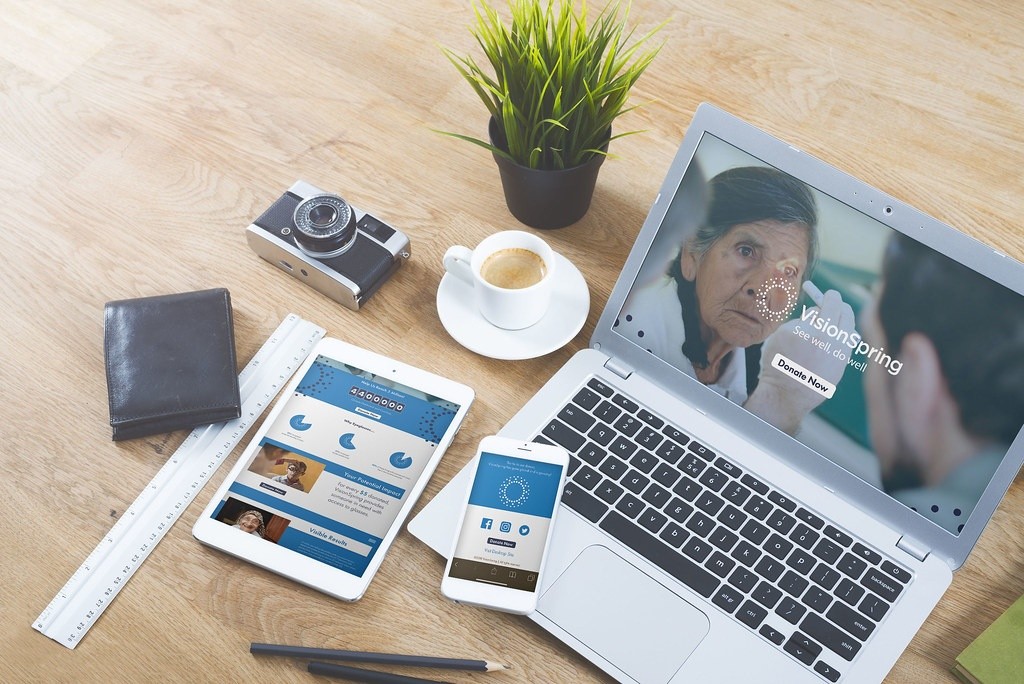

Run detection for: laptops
[[406, 104, 1024, 684]]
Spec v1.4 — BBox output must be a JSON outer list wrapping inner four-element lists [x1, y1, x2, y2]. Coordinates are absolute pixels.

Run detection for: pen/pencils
[[250, 643, 512, 674], [308, 661, 455, 684]]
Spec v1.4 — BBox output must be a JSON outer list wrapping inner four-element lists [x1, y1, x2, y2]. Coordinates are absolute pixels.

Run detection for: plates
[[436, 250, 590, 360]]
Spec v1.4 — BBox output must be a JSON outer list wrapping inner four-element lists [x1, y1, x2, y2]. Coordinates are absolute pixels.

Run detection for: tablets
[[190, 335, 475, 602]]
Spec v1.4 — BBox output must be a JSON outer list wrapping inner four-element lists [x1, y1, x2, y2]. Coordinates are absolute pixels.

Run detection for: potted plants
[[436, 0, 683, 228]]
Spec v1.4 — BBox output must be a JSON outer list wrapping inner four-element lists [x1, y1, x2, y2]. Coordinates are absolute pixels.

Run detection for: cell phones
[[441, 436, 569, 615]]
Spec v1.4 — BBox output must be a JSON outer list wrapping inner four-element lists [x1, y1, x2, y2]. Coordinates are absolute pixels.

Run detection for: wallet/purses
[[103, 288, 242, 440]]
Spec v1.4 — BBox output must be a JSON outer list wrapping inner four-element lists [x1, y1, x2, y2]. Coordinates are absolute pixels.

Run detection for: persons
[[861, 230, 1024, 535], [248, 443, 307, 491], [232, 510, 264, 539], [613, 166, 855, 436]]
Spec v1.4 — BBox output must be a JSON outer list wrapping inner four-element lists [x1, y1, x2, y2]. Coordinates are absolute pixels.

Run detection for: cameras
[[246, 181, 411, 312]]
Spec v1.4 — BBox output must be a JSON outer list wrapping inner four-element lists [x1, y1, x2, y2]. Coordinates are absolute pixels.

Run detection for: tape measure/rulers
[[31, 313, 327, 649]]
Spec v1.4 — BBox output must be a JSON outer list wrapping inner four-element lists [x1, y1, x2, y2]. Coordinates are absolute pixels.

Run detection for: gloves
[[743, 289, 860, 436]]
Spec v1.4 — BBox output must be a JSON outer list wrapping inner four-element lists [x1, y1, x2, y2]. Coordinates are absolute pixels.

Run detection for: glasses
[[288, 467, 305, 476]]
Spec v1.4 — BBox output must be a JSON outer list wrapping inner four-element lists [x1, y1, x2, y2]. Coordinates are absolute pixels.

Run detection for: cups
[[442, 230, 555, 330]]
